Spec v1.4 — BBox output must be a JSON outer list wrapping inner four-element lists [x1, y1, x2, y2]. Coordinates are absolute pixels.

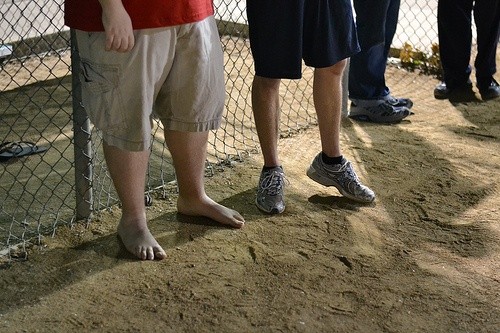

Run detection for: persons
[[64, 0, 245, 262], [347, 0, 413, 123], [244, 0, 376, 214], [433, 0, 500, 100]]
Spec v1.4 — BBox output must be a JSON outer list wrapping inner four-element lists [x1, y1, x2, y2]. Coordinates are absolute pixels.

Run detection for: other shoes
[[479, 80, 500, 101], [434, 83, 475, 99]]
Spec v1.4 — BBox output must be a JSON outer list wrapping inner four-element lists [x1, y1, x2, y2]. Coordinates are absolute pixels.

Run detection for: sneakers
[[380, 94, 413, 109], [255, 165, 286, 214], [348, 97, 409, 122], [306, 150, 375, 202]]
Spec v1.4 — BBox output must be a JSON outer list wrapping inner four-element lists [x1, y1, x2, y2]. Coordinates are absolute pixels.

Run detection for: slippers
[[0, 141, 49, 158]]
